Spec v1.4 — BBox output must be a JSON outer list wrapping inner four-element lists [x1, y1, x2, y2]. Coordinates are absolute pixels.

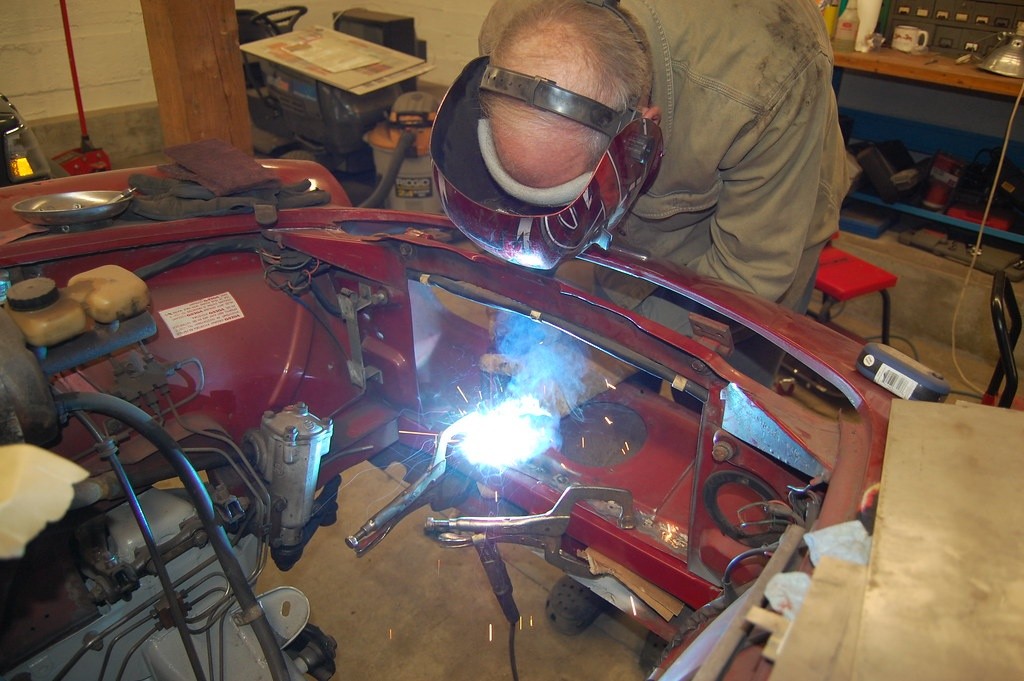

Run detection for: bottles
[[832, 0, 860, 52]]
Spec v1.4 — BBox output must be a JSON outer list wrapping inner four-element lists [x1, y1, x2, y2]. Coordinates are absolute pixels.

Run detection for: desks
[[828, 39, 1024, 243]]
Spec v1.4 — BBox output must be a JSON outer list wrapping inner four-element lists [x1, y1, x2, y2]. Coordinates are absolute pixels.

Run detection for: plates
[[11, 190, 139, 224]]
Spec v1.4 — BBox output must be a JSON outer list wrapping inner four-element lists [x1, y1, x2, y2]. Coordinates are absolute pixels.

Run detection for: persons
[[479, 1, 858, 671]]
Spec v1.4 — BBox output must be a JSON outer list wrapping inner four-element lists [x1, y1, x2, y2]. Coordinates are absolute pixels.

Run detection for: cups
[[891, 24, 929, 53]]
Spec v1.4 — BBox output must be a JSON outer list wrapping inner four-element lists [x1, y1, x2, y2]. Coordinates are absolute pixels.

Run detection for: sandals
[[545, 576, 608, 636], [640, 631, 669, 669]]
